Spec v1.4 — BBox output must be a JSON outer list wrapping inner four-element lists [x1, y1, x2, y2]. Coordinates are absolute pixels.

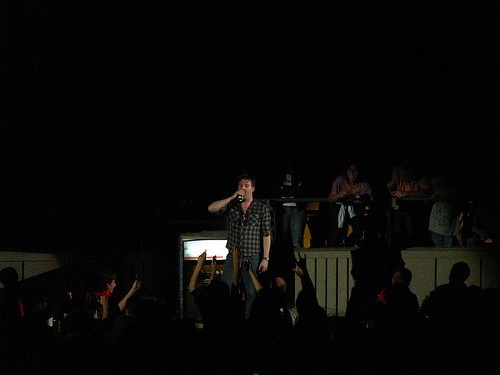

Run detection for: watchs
[[263, 257, 269, 261]]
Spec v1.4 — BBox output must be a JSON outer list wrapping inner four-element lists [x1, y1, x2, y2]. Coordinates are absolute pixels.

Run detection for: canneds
[[238, 195, 244, 201]]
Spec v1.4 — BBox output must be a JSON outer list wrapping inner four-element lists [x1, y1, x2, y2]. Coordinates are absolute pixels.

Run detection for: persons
[[327, 164, 374, 246], [279, 162, 308, 250], [386, 163, 419, 246], [1, 241, 500, 375], [208, 178, 271, 312]]
